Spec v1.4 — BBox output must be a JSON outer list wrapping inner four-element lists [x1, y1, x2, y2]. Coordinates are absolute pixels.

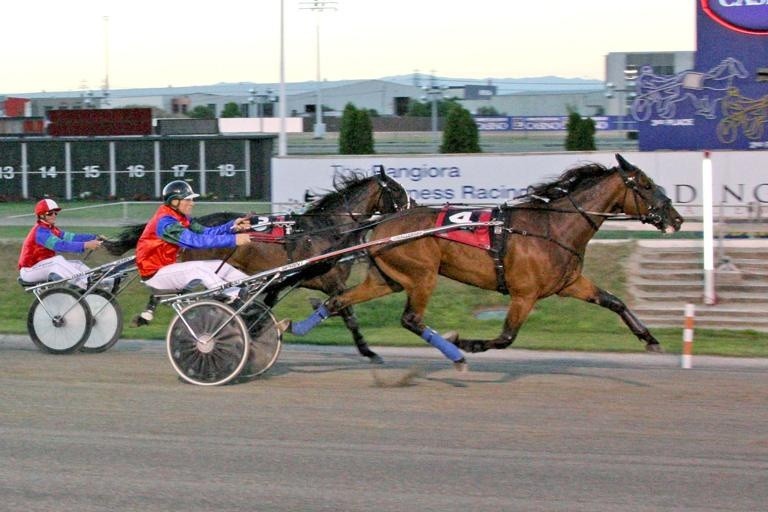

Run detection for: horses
[[95, 165, 419, 365], [264, 153, 684, 373]]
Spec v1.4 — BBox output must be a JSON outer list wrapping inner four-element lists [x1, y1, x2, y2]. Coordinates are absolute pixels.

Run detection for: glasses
[[45, 212, 58, 216]]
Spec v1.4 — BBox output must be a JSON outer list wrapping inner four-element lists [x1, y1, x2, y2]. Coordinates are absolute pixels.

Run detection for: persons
[[14, 197, 123, 293], [136, 177, 265, 314], [304, 189, 315, 202]]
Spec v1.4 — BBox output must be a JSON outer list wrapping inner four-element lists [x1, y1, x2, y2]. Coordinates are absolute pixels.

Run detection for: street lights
[[249, 88, 279, 132], [421, 83, 451, 146], [298, 0, 341, 137], [605, 81, 637, 145]]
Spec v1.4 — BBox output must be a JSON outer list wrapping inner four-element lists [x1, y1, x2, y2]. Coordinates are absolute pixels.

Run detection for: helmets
[[34, 198, 61, 216], [162, 179, 201, 203]]
